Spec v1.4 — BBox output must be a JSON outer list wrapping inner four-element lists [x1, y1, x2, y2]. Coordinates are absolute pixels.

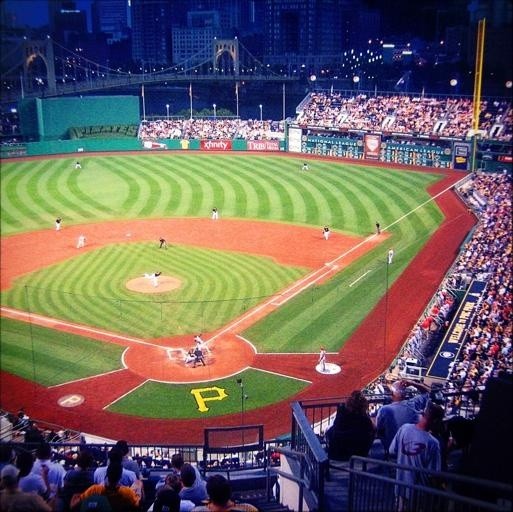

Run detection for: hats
[[1, 465, 20, 479]]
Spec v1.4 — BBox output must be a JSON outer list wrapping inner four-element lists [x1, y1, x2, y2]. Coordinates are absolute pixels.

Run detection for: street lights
[[213, 104, 216, 121], [259, 105, 263, 120], [166, 104, 170, 119]]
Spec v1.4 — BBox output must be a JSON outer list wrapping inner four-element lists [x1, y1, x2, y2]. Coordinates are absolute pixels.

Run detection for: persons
[[141, 271, 161, 288], [54, 216, 63, 231], [184, 333, 211, 368], [136, 91, 512, 167], [0, 388, 513, 511], [301, 160, 309, 171], [159, 237, 167, 250], [212, 206, 218, 220], [75, 160, 81, 168], [321, 225, 330, 240], [316, 347, 326, 372], [76, 233, 86, 248], [367, 166, 512, 388]]
